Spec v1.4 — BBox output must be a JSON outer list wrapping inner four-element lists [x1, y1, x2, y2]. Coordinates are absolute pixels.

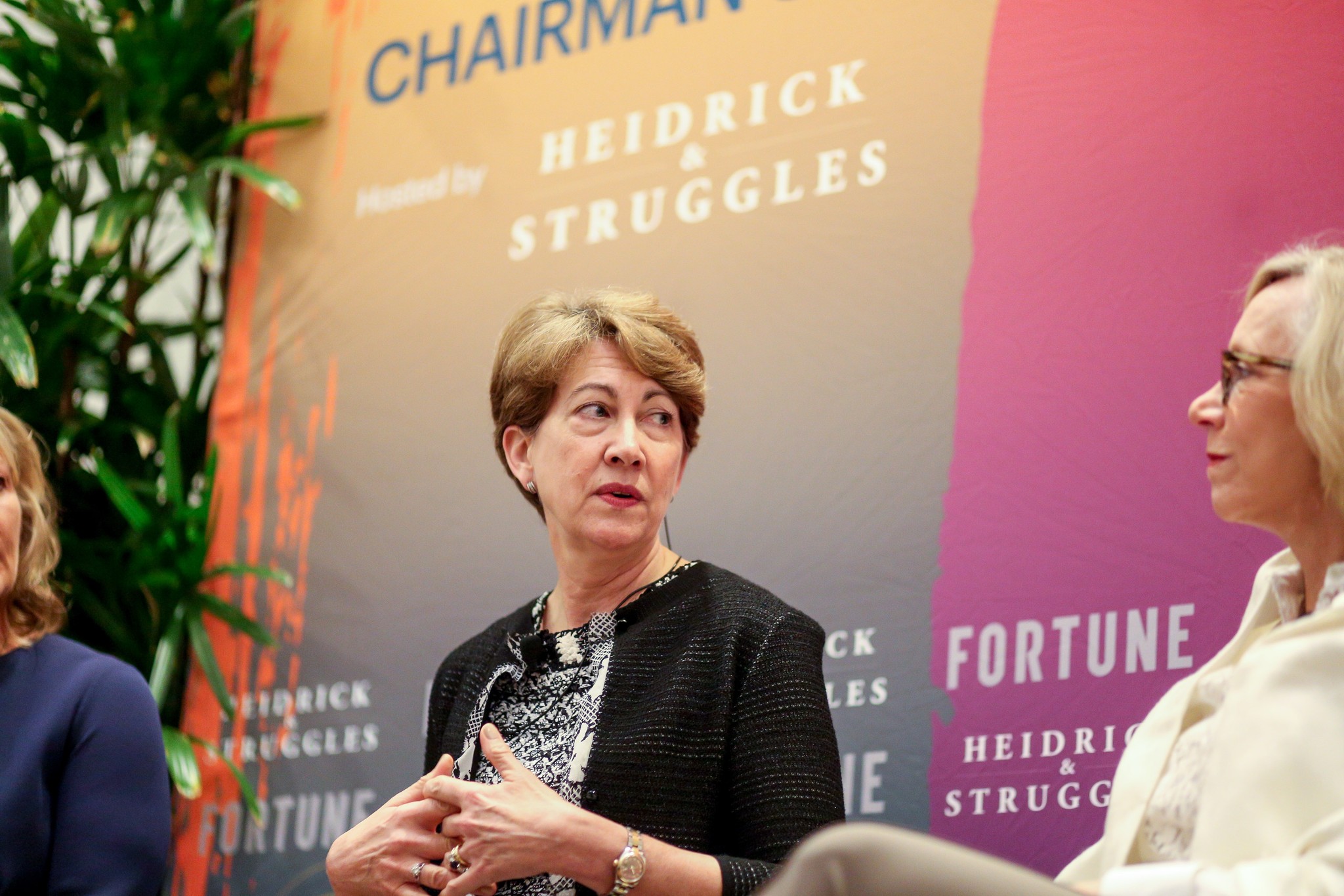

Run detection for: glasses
[[1220, 346, 1291, 408]]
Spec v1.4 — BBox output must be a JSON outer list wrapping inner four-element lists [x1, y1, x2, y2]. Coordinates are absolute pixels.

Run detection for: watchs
[[609, 827, 646, 896]]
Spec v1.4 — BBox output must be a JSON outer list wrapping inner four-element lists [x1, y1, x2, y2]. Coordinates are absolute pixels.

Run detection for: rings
[[411, 860, 425, 882], [449, 844, 468, 873]]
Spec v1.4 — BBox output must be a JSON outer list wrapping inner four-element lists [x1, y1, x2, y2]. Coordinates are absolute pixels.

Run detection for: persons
[[767, 246, 1344, 896], [0, 408, 174, 896], [327, 291, 845, 896]]
